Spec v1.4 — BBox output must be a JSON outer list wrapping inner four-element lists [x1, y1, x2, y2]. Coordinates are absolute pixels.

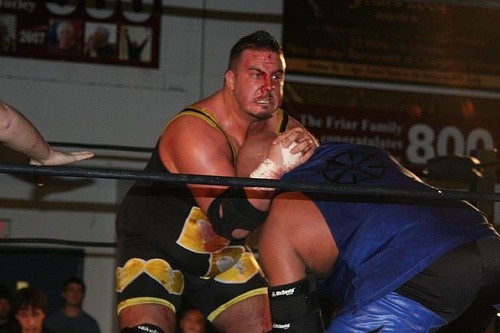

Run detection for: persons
[[84, 26, 112, 63], [0, 99, 95, 166], [49, 19, 80, 58], [124, 28, 149, 61], [116, 30, 500, 333], [0, 19, 12, 52], [0, 274, 102, 333]]
